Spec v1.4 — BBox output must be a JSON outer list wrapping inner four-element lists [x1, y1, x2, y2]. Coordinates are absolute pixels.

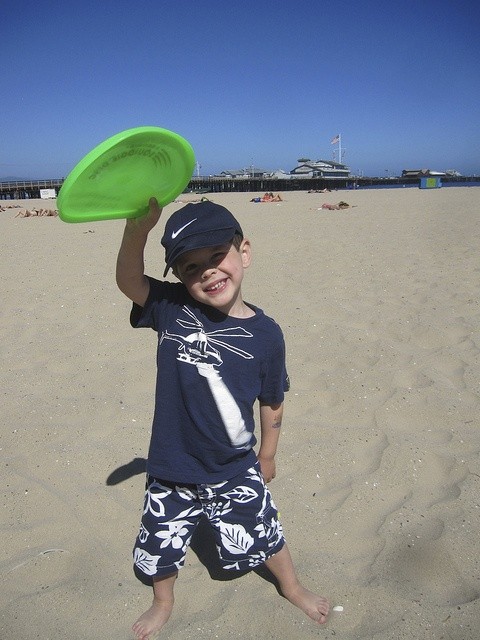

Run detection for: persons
[[252, 190, 282, 205], [116, 198, 330, 640], [313, 200, 350, 209], [0, 203, 59, 218]]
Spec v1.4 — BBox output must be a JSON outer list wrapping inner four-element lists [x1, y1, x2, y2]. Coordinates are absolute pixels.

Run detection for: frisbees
[[57, 126, 197, 223]]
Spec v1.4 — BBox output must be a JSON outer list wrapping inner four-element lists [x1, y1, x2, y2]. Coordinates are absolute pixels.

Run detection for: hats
[[160, 198, 244, 277]]
